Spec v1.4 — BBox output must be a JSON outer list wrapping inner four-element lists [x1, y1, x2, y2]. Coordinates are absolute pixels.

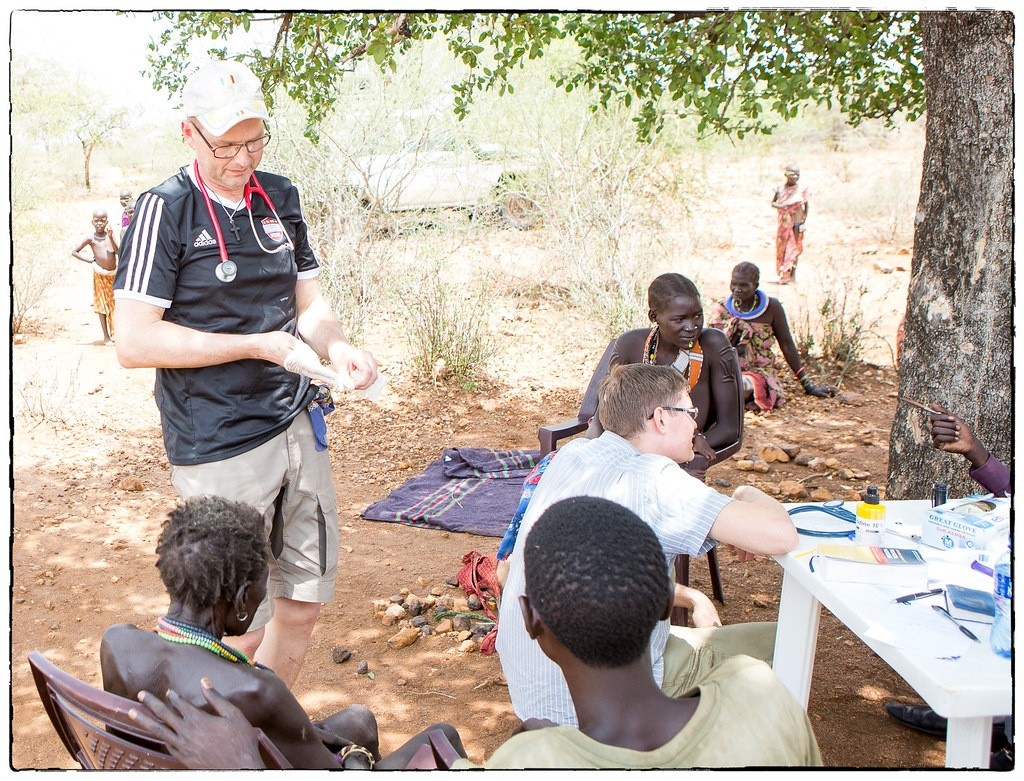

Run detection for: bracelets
[[796, 367, 810, 388], [691, 433, 707, 441], [337, 745, 376, 769]]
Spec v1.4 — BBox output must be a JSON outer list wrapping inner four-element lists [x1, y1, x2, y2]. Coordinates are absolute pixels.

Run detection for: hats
[[185, 60, 270, 136]]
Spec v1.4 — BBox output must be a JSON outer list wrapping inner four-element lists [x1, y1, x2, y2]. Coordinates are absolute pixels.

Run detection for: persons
[[494, 272, 740, 589], [771, 163, 809, 285], [885, 404, 1015, 772], [128, 495, 822, 769], [99, 494, 467, 772], [72, 211, 120, 345], [126, 199, 137, 221], [120, 188, 135, 227], [711, 261, 836, 402], [112, 60, 376, 689], [495, 364, 798, 728]]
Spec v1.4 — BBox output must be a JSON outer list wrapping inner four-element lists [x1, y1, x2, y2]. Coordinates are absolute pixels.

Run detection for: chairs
[[28, 650, 294, 770], [538, 347, 743, 628]]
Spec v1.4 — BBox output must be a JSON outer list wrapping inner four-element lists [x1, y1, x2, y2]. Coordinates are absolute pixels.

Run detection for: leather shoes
[[884, 701, 947, 742]]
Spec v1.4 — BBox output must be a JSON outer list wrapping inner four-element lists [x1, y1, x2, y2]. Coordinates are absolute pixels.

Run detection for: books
[[943, 585, 995, 624]]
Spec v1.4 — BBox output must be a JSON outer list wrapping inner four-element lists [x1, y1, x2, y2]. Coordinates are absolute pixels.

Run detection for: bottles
[[932, 484, 947, 508], [989, 535, 1011, 660], [855, 485, 887, 547]]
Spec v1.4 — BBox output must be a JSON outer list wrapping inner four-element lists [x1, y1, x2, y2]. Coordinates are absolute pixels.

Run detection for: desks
[[769, 496, 1015, 770]]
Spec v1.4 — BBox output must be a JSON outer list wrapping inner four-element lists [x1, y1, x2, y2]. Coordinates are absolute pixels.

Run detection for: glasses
[[647, 407, 700, 421], [190, 121, 272, 159]]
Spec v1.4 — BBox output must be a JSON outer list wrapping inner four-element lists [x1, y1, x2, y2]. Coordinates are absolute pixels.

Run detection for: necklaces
[[151, 615, 274, 671], [93, 230, 108, 240], [202, 181, 245, 241], [643, 325, 703, 392], [724, 289, 769, 320]]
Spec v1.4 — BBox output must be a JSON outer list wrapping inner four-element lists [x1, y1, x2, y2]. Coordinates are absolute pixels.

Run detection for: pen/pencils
[[931, 604, 981, 644], [899, 397, 942, 414], [890, 588, 943, 604]]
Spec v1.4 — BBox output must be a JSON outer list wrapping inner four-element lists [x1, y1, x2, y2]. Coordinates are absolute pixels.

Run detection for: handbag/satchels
[[459, 551, 502, 656]]
